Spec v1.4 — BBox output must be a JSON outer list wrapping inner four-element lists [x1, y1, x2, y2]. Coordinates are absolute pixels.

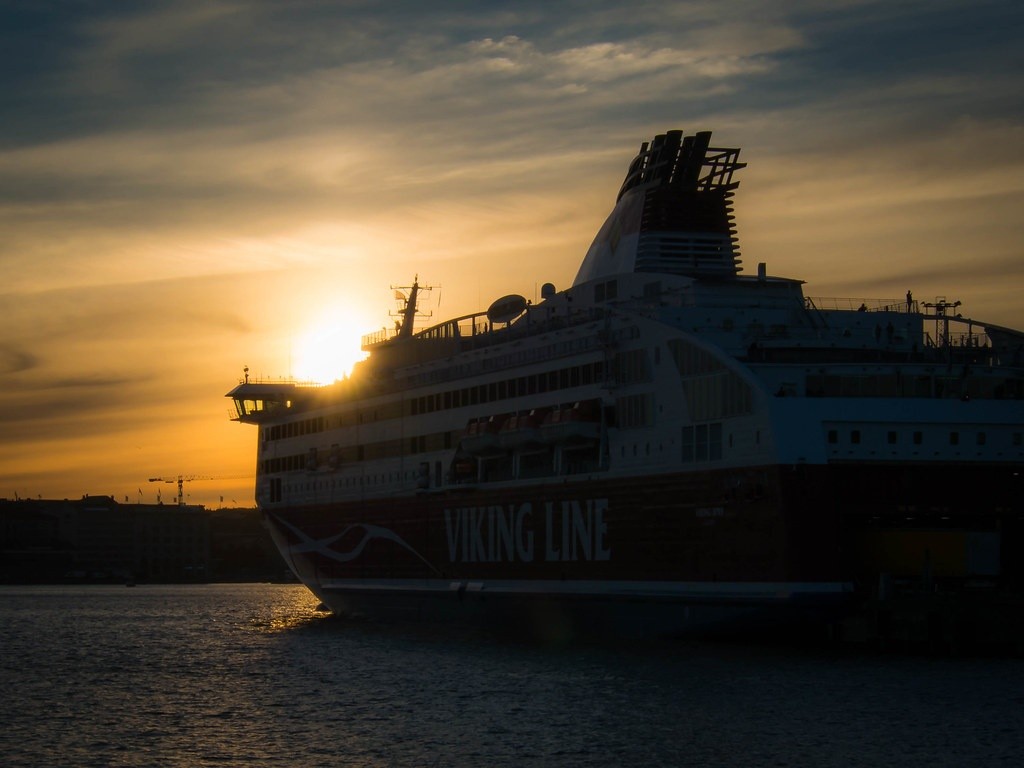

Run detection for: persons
[[963, 391, 969, 398], [875, 322, 882, 344], [907, 290, 912, 313], [886, 322, 894, 345], [858, 303, 867, 311], [776, 387, 785, 397]]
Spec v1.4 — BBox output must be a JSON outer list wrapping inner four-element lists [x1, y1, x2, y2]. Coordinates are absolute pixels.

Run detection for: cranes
[[148, 475, 254, 507]]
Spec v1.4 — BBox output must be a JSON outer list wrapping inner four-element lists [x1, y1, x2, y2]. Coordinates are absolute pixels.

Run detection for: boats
[[230, 129, 1024, 645]]
